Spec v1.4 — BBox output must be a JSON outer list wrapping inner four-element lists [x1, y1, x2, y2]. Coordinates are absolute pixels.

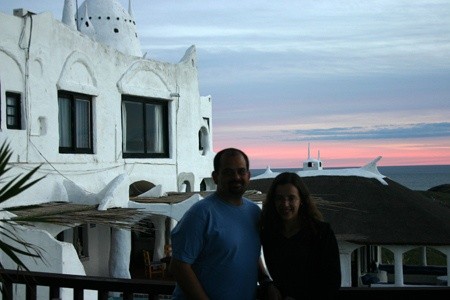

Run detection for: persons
[[257, 171, 342, 300], [170, 148, 265, 300]]
